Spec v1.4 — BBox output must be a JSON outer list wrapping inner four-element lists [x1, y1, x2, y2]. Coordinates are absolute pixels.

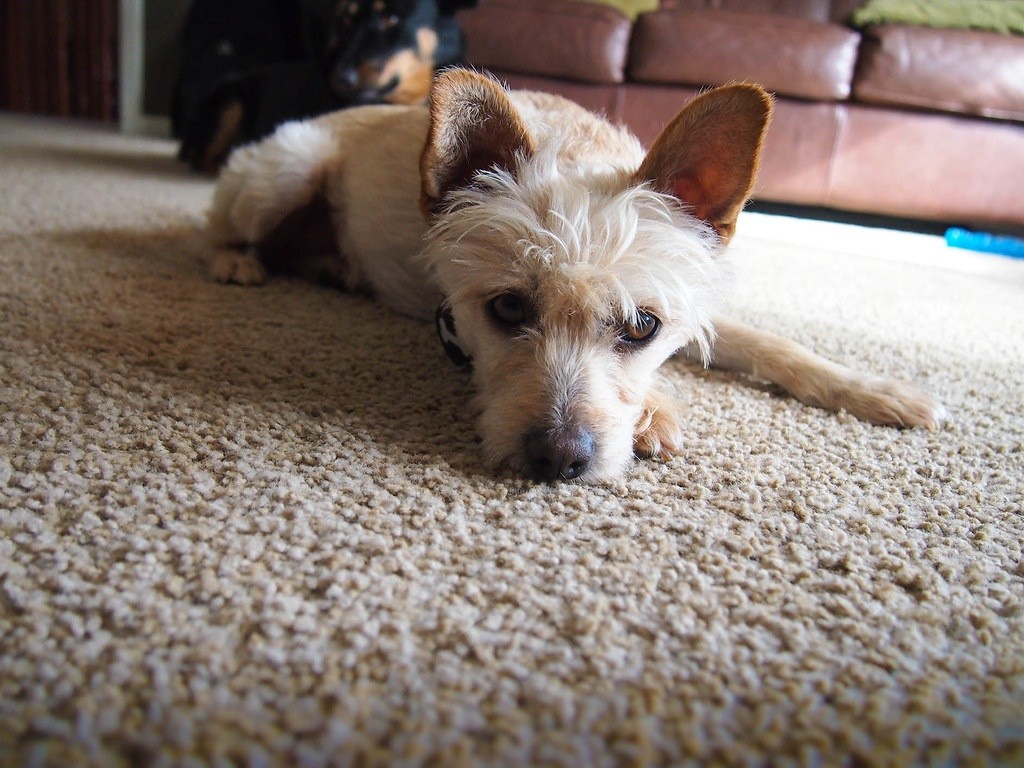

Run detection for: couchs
[[454, 0, 1024, 224]]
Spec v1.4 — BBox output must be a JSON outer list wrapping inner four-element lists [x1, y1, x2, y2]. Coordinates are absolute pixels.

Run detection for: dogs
[[206, 66, 950, 488], [162, 0, 481, 183]]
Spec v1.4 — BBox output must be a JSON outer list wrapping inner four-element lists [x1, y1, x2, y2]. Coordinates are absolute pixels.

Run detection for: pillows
[[853, 0, 1024, 34]]
